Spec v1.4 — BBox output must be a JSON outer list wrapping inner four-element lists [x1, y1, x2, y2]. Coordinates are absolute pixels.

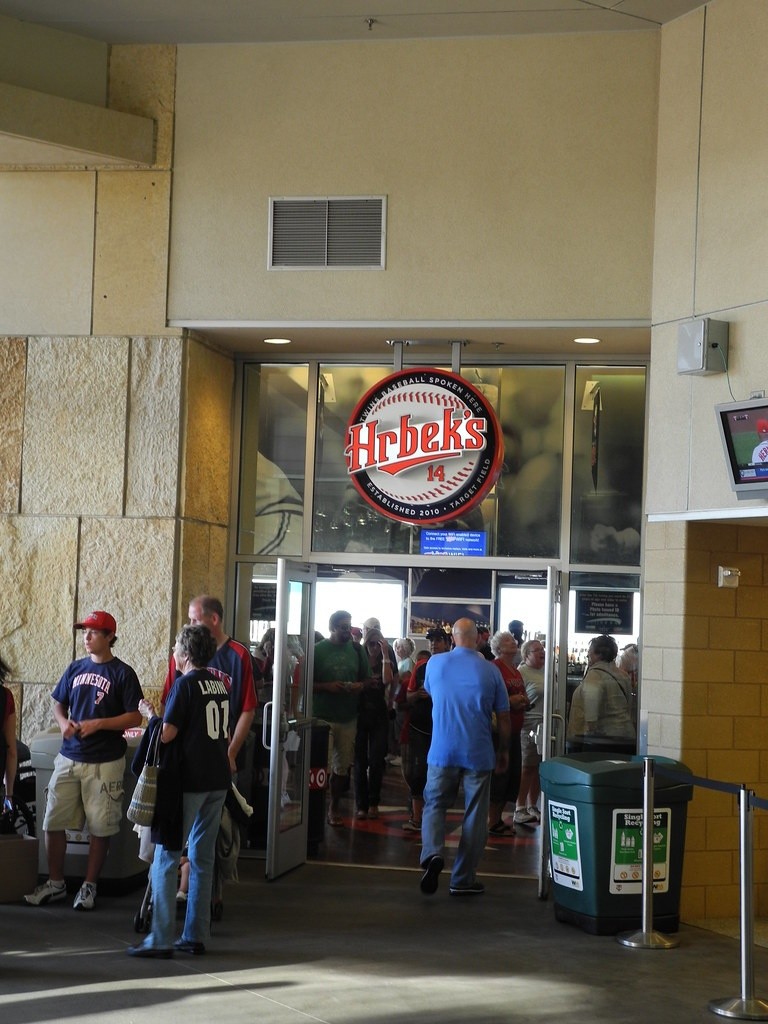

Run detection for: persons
[[249, 610, 495, 831], [176, 841, 190, 901], [566, 636, 637, 754], [126, 627, 232, 959], [0, 660, 18, 834], [487, 632, 530, 835], [160, 595, 257, 774], [23, 611, 144, 910], [419, 618, 511, 896], [513, 640, 558, 824], [509, 620, 525, 645]]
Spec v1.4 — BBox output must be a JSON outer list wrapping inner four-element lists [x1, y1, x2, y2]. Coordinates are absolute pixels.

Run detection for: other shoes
[[449, 878, 485, 891], [176, 891, 185, 900], [367, 808, 378, 818], [357, 811, 365, 819], [173, 936, 205, 955], [402, 821, 421, 830], [328, 812, 344, 825], [419, 854, 444, 896], [127, 941, 176, 959]]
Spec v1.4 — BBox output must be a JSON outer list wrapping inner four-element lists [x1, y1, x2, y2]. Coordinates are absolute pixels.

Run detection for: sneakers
[[513, 808, 537, 824], [23, 881, 67, 905], [528, 806, 541, 822], [73, 881, 97, 909]]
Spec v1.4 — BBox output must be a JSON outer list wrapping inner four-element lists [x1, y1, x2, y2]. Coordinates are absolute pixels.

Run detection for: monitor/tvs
[[714, 397, 768, 492]]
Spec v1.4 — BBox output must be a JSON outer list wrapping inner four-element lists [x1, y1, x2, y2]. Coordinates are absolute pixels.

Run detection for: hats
[[73, 611, 116, 634]]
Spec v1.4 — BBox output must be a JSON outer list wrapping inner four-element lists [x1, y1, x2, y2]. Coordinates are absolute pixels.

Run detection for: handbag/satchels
[[127, 723, 161, 827]]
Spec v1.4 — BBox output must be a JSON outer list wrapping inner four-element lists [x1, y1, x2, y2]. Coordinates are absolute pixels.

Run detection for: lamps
[[717, 565, 741, 589]]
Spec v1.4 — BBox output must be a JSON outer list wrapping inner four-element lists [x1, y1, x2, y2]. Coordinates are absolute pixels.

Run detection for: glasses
[[334, 624, 351, 630], [528, 648, 544, 653], [368, 641, 380, 646], [172, 646, 184, 651]]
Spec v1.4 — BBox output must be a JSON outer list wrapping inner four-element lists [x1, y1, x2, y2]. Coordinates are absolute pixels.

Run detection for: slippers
[[486, 824, 513, 837], [499, 820, 517, 834]]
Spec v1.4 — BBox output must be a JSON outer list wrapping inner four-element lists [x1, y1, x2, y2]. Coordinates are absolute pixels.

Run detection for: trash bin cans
[[539, 751, 694, 936], [31, 726, 152, 897]]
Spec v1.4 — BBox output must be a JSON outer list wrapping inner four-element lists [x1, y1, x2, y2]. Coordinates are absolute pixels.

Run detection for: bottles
[[570, 648, 588, 664], [413, 617, 489, 638]]
